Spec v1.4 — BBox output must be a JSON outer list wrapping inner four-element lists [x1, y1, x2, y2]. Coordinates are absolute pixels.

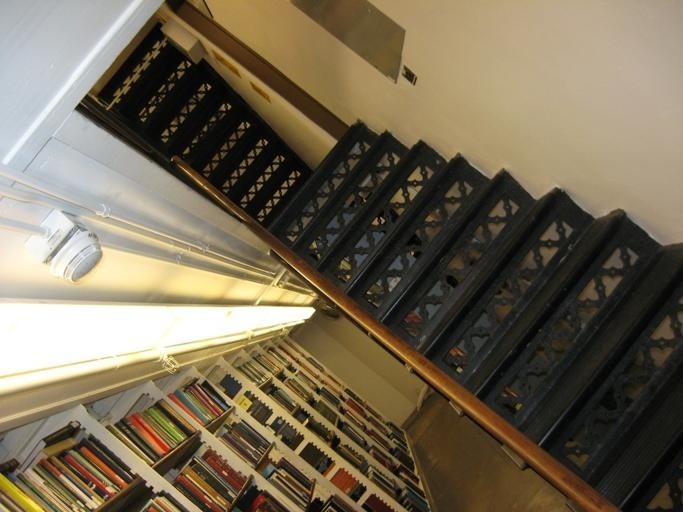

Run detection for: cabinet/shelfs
[[0, 334, 437, 512]]
